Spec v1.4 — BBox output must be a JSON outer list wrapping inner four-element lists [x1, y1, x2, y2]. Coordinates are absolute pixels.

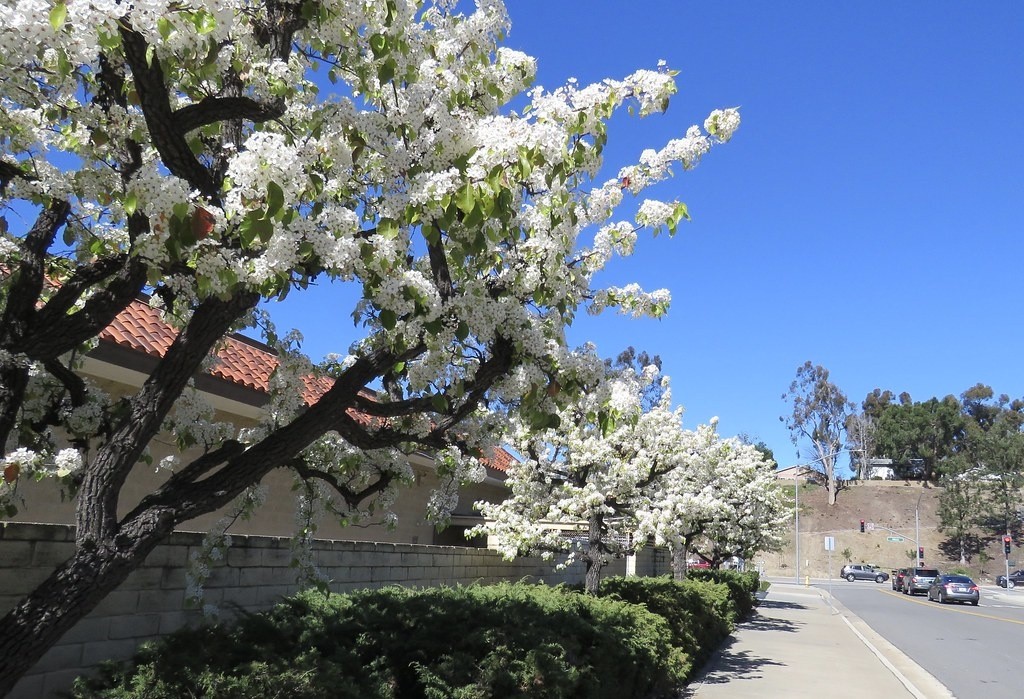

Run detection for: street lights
[[796, 449, 862, 584], [915, 491, 925, 566]]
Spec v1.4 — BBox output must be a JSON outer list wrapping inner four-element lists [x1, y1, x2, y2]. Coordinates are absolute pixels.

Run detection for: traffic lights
[[919, 547, 923, 558], [861, 520, 864, 533], [1003, 537, 1011, 553]]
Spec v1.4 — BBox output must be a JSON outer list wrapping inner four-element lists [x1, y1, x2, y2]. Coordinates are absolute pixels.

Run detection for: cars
[[839, 564, 889, 583], [891, 573, 896, 590], [928, 575, 979, 605], [903, 567, 940, 595], [996, 569, 1024, 589], [687, 556, 743, 570], [892, 568, 907, 592]]
